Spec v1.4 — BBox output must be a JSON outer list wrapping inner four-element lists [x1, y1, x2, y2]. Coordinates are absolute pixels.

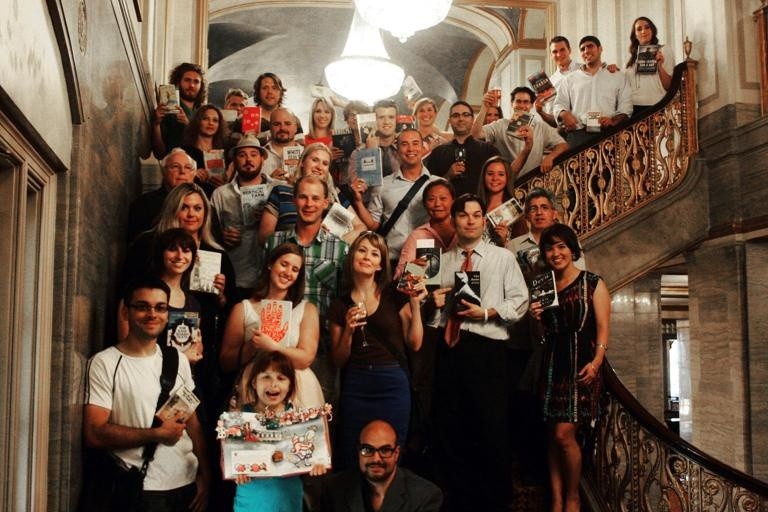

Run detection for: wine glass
[[348, 302, 372, 349]]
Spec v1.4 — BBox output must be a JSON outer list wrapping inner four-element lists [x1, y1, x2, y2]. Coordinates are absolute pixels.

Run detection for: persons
[[605, 16, 675, 120], [330, 231, 428, 467], [424, 193, 528, 512], [529, 225, 612, 512], [218, 351, 327, 512], [552, 36, 634, 145], [348, 420, 440, 512], [83, 276, 212, 511], [534, 36, 586, 141], [118, 63, 570, 415]]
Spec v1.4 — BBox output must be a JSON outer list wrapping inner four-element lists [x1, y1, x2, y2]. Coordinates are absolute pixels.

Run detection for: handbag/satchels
[[75, 447, 144, 511]]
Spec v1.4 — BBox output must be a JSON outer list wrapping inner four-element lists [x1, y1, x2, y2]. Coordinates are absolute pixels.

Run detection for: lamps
[[324, 0, 452, 104]]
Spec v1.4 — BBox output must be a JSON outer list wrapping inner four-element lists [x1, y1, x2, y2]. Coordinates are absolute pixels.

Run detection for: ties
[[444, 249, 474, 348]]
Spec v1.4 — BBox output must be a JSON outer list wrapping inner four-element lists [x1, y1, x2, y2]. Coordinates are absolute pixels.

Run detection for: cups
[[492, 88, 501, 107], [455, 148, 466, 163]]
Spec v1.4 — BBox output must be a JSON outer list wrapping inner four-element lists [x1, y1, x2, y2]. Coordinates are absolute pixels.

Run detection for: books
[[218, 403, 332, 480], [156, 386, 201, 423], [586, 111, 602, 134], [637, 42, 659, 73]]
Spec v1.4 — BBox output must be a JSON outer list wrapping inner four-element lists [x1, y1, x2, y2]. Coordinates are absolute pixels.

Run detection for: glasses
[[450, 112, 473, 119], [360, 445, 398, 459]]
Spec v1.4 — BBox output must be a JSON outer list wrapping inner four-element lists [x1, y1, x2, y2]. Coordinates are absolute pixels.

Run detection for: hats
[[228, 133, 268, 161]]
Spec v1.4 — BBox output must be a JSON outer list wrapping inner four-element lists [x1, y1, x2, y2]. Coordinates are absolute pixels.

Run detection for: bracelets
[[590, 362, 596, 373], [595, 342, 610, 353]]
